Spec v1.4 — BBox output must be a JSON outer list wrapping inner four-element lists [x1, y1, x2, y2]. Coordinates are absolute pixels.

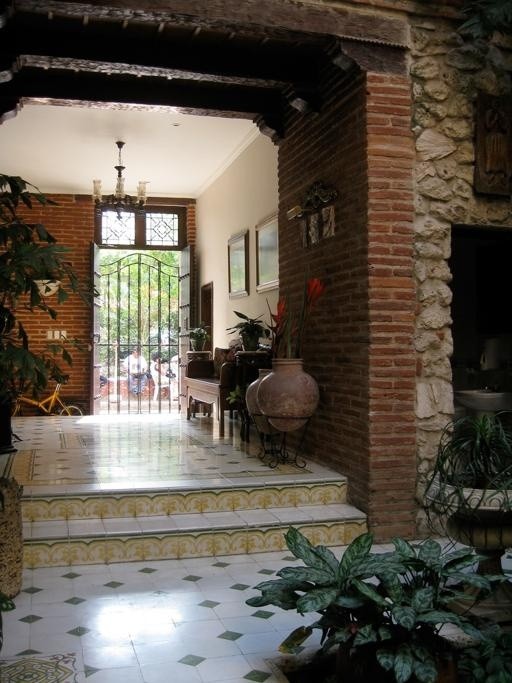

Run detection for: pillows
[[212, 344, 239, 379]]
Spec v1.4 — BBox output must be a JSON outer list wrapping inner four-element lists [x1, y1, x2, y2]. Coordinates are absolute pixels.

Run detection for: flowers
[[263, 274, 327, 359]]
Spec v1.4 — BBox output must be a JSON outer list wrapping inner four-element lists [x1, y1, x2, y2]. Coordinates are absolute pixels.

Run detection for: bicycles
[[9, 372, 85, 416]]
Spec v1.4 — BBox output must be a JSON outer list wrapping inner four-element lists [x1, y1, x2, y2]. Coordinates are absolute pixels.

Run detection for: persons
[[99, 374, 114, 398], [123, 346, 147, 395], [151, 351, 180, 403]]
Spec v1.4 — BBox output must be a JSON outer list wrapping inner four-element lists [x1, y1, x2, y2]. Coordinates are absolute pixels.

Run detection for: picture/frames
[[226, 227, 250, 302], [253, 211, 279, 295]]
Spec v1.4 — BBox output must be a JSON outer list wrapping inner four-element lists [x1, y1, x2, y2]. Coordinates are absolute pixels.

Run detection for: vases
[[254, 361, 322, 435], [245, 367, 276, 437]]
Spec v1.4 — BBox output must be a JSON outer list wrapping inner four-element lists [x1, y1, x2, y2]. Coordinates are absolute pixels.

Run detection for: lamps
[[284, 179, 342, 224], [90, 140, 151, 223]]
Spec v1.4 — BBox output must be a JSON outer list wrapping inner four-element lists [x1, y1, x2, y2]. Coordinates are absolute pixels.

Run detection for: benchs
[[180, 357, 236, 439]]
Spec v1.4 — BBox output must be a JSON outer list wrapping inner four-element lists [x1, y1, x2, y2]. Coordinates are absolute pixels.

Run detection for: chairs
[[148, 360, 170, 401]]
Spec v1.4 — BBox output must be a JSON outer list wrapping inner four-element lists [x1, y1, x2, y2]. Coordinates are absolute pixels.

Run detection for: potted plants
[[176, 319, 214, 351], [226, 309, 270, 352], [0, 168, 103, 460], [416, 409, 512, 581]]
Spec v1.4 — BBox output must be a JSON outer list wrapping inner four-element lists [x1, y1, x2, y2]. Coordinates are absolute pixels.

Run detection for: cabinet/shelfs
[[235, 350, 272, 442], [185, 349, 213, 418]]
